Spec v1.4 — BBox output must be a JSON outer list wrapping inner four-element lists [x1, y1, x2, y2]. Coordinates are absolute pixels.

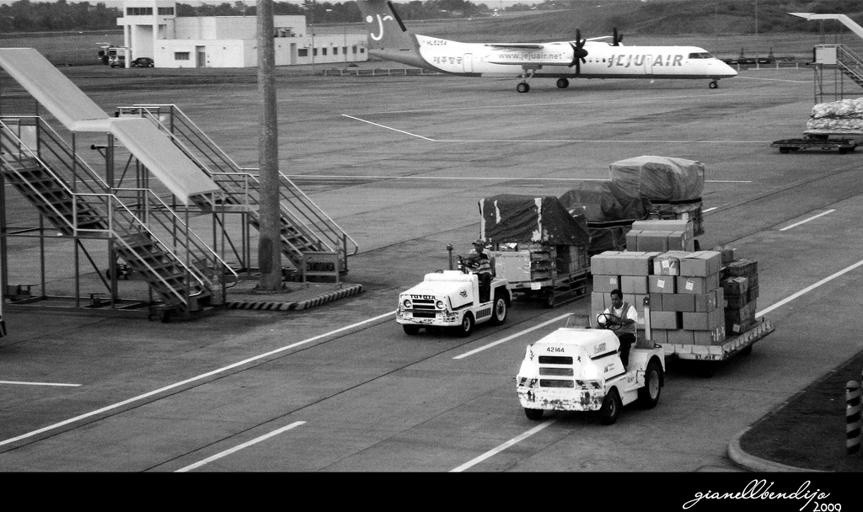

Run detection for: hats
[[471, 239, 485, 246]]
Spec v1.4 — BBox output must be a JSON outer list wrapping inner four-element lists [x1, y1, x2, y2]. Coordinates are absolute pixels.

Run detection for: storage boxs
[[589, 218, 729, 346]]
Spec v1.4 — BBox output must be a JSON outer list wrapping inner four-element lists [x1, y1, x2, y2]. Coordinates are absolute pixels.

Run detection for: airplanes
[[350, 0, 743, 97]]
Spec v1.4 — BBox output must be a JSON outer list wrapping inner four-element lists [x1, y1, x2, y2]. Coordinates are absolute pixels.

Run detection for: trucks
[[94, 40, 113, 64], [107, 46, 125, 69]]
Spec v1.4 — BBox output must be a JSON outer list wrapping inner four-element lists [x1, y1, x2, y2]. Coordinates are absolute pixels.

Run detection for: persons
[[465, 239, 491, 303], [595, 288, 638, 371]]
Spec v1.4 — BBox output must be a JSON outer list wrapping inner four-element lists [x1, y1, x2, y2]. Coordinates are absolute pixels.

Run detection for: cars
[[129, 57, 155, 68]]
